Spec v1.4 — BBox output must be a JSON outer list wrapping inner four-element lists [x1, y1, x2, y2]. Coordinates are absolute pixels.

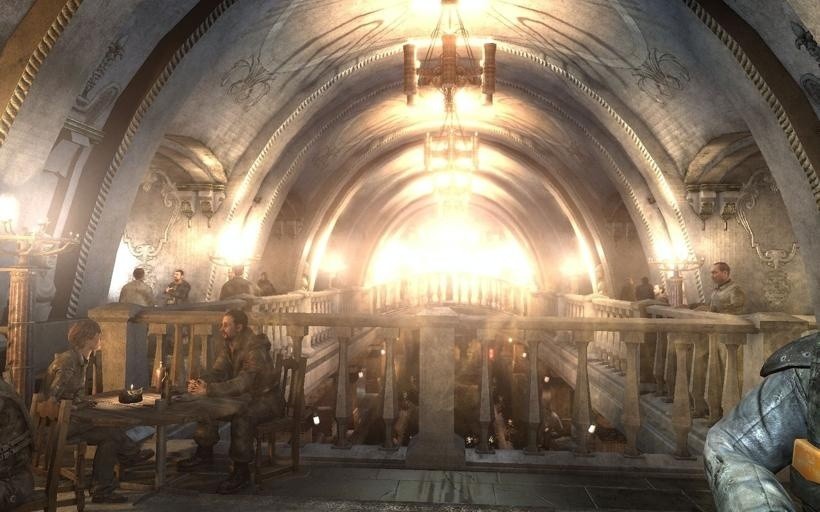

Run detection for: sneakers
[[138, 447, 154, 461], [93, 492, 128, 504]]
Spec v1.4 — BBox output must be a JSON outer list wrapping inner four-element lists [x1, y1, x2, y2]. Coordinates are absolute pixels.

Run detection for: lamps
[[403, 0, 497, 177]]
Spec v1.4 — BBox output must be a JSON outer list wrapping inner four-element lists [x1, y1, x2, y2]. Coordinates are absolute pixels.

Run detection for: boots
[[178, 445, 213, 471], [218, 461, 250, 494]]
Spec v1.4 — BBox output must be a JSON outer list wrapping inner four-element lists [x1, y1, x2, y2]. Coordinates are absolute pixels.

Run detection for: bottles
[[155, 360, 172, 399]]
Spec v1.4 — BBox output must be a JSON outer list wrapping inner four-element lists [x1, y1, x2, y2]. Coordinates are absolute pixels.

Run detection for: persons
[[537, 408, 564, 451], [1, 269, 288, 512], [703, 332, 820, 512], [621, 277, 668, 304], [676, 261, 745, 314]]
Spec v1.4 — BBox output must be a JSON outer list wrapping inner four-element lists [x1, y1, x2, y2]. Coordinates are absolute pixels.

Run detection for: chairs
[[26, 333, 308, 512]]
